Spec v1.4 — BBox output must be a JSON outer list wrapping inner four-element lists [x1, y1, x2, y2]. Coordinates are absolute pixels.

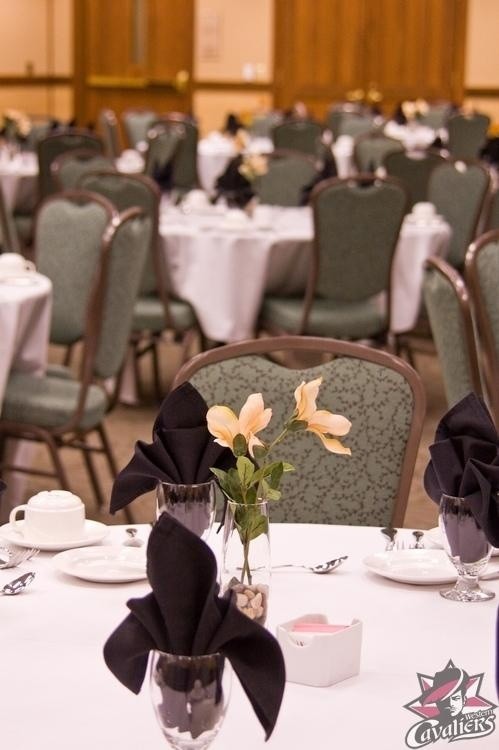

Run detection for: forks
[[407, 530, 426, 550], [0, 541, 40, 570], [381, 526, 406, 551]]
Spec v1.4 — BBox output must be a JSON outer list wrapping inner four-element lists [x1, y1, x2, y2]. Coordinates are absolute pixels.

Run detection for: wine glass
[[436, 494, 496, 603]]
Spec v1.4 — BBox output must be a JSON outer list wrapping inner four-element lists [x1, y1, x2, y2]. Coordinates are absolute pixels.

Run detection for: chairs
[[0, 87, 499, 750]]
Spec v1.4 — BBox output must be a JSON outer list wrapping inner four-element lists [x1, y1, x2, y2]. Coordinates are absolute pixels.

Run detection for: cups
[[148, 645, 232, 750], [0, 259, 36, 278], [152, 481, 217, 543], [9, 502, 86, 539], [414, 202, 438, 220]]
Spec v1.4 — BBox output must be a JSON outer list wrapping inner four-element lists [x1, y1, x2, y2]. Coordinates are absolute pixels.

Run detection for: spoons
[[0, 570, 36, 596], [122, 526, 144, 547], [237, 554, 350, 574]]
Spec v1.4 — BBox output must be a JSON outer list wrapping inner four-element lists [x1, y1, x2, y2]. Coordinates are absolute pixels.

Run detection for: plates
[[0, 274, 39, 288], [0, 514, 112, 551], [361, 546, 499, 585], [155, 188, 314, 234], [49, 543, 149, 585], [426, 526, 499, 556]]
